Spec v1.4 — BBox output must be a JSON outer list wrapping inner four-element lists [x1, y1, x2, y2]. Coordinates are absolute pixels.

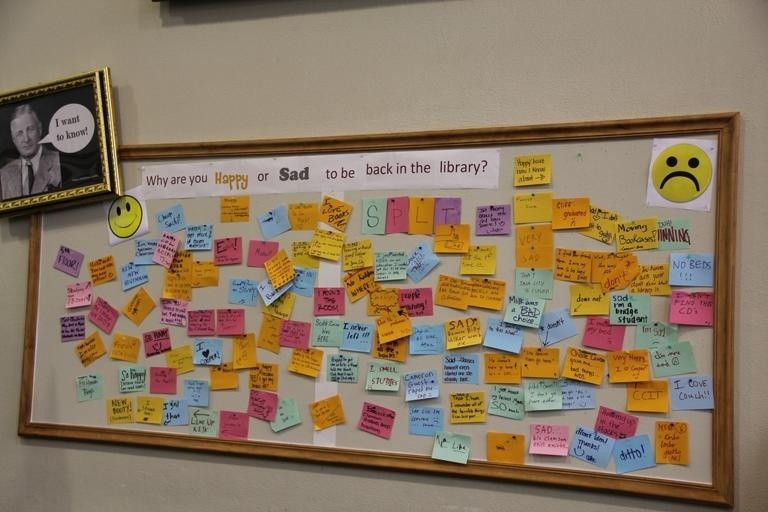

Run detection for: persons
[[0, 104, 63, 201]]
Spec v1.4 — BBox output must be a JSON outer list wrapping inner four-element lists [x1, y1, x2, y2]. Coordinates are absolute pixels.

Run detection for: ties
[[28, 162, 35, 193]]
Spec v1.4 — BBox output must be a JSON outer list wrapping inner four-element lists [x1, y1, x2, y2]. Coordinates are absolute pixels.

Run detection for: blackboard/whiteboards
[[17, 111, 741, 508]]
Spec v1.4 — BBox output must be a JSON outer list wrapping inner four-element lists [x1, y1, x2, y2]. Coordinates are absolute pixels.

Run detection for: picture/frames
[[0, 66, 122, 222]]
